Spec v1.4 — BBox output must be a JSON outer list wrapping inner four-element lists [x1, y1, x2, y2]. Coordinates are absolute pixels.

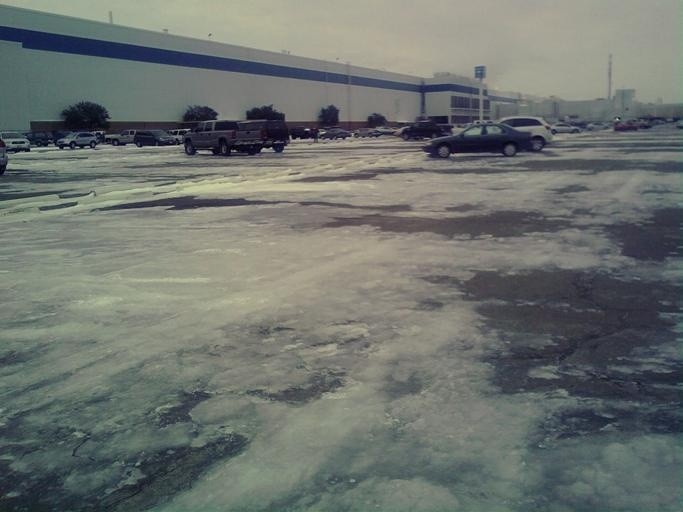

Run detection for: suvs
[[234, 118, 291, 154]]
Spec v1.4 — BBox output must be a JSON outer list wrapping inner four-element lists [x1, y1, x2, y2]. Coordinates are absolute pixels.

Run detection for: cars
[[400, 120, 442, 141], [0, 131, 31, 153], [104, 127, 191, 147], [496, 115, 555, 153], [56, 131, 100, 149], [571, 115, 683, 132], [421, 120, 535, 161], [550, 121, 583, 135], [21, 130, 55, 147], [291, 122, 411, 143]]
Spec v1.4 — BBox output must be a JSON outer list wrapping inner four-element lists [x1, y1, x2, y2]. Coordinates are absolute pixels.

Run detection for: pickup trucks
[[182, 118, 268, 157]]
